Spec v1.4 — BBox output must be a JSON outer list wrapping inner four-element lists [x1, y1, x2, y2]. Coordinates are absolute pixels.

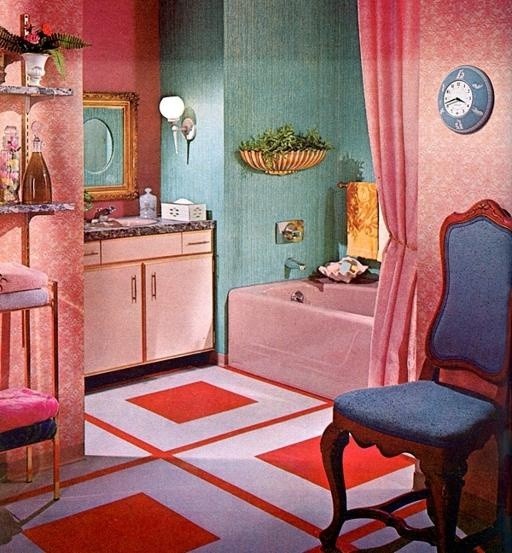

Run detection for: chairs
[[319, 198, 511, 553]]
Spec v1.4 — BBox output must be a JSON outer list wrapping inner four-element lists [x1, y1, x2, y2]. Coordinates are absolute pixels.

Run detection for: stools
[[1, 386, 60, 502]]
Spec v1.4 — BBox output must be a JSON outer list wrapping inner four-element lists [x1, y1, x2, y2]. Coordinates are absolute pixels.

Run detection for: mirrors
[[82, 91, 140, 202]]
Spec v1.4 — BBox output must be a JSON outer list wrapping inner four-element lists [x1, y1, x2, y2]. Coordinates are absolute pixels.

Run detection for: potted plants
[[0, 26, 93, 89], [238, 122, 335, 175]]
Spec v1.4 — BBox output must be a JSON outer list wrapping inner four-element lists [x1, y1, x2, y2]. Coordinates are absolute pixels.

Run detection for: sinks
[[88, 219, 158, 228]]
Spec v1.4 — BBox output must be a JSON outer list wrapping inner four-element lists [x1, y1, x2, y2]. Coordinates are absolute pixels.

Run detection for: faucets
[[93, 206, 116, 219], [286, 258, 307, 272]]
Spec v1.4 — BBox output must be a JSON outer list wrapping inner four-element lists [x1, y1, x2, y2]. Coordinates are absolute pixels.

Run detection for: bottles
[[22, 138, 51, 206]]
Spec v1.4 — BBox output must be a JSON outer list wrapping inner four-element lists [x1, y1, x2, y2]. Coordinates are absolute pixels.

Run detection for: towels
[[1, 386, 60, 451], [1, 264, 49, 311]]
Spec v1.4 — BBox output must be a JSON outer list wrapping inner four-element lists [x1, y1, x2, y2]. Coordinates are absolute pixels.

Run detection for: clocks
[[437, 65, 494, 134]]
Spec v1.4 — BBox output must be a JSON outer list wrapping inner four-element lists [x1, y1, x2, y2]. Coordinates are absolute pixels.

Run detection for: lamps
[[159, 96, 195, 154]]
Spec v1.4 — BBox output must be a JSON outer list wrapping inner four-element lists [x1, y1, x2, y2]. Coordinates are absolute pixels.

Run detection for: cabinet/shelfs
[[83, 229, 216, 377], [0, 278, 59, 422]]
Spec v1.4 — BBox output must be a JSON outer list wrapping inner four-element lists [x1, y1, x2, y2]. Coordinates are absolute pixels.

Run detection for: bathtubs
[[229, 279, 377, 402]]
